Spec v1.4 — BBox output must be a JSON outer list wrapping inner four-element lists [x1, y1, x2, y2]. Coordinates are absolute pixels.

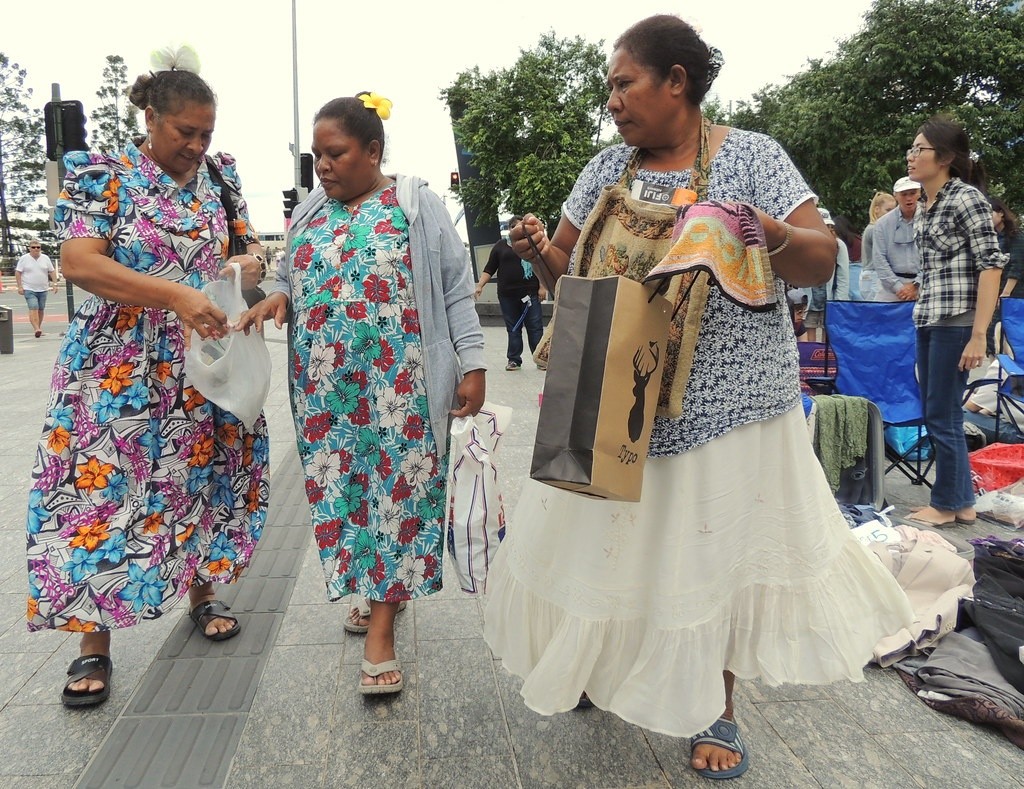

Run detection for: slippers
[[188, 600, 242, 640], [59, 653, 115, 707], [344, 596, 411, 634], [905, 513, 957, 530], [359, 658, 405, 697], [954, 518, 976, 526]]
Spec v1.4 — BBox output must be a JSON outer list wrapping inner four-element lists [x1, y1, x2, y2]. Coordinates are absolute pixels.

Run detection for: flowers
[[359, 91, 393, 120], [149, 44, 201, 74]]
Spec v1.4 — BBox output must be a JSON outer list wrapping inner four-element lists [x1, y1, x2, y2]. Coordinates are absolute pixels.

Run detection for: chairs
[[993, 295, 1024, 454], [804, 297, 1001, 490]]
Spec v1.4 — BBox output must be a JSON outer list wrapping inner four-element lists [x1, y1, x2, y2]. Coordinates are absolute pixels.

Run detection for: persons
[[475, 215, 547, 371], [266, 245, 280, 270], [15, 240, 58, 338], [236, 91, 487, 694], [907, 118, 1010, 529], [26, 70, 270, 706], [483, 13, 915, 779], [786, 176, 1024, 342]]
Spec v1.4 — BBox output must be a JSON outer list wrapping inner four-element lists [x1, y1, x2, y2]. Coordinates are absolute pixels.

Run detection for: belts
[[894, 272, 918, 279]]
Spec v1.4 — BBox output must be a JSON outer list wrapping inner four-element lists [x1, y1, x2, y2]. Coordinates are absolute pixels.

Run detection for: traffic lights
[[283, 189, 297, 219]]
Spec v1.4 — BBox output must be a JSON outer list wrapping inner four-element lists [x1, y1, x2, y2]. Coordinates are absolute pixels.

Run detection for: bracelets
[[768, 222, 792, 257], [248, 253, 266, 284]]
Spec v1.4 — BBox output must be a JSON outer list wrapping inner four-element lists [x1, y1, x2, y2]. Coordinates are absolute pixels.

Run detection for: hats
[[893, 177, 923, 192], [816, 208, 835, 226], [786, 288, 810, 304]]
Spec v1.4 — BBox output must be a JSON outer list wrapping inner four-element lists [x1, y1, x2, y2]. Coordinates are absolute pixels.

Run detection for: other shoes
[[505, 361, 522, 371], [536, 365, 547, 371], [35, 330, 42, 338]]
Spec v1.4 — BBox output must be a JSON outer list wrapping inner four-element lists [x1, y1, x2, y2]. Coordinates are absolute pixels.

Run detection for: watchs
[[912, 281, 920, 288]]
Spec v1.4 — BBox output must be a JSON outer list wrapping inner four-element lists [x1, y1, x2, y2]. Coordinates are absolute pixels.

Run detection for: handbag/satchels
[[520, 215, 704, 504], [204, 154, 268, 309], [176, 261, 273, 431], [527, 116, 711, 425], [448, 401, 516, 599]]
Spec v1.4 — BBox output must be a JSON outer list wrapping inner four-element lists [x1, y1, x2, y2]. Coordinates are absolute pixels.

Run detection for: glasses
[[31, 246, 40, 249], [906, 147, 936, 158]]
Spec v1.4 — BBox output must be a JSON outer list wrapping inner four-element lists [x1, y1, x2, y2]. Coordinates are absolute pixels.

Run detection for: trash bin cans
[[0, 305, 14, 354]]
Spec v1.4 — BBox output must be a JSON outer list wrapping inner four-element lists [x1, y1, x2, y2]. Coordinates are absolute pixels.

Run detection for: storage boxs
[[885, 515, 975, 574]]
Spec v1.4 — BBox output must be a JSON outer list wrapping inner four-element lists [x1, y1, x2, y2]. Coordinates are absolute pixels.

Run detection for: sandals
[[691, 717, 749, 779]]
